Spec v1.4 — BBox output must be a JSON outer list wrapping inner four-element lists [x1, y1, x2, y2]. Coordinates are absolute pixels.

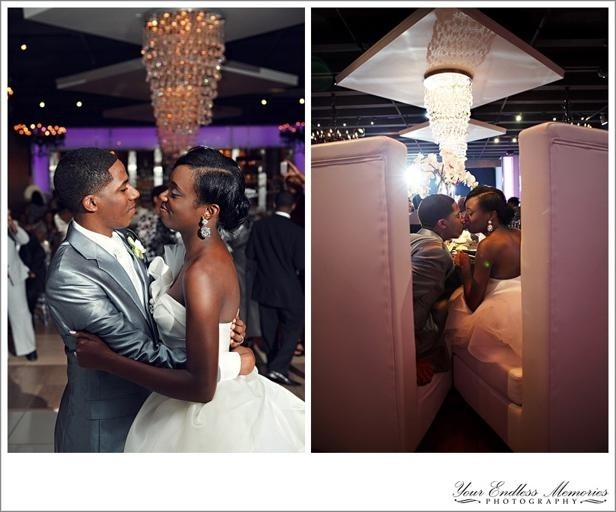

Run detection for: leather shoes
[[25, 351, 38, 360], [265, 369, 301, 386]]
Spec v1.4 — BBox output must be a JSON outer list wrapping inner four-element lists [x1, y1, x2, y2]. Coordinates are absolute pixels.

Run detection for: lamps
[[423, 71, 474, 185], [142, 8, 226, 163]]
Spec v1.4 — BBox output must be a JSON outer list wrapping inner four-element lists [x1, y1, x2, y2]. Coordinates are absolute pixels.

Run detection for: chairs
[[311, 135, 452, 452], [452, 121, 608, 452]]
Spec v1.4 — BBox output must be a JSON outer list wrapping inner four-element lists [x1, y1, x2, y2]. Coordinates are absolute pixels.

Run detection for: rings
[[240, 336, 245, 343], [74, 352, 77, 358]]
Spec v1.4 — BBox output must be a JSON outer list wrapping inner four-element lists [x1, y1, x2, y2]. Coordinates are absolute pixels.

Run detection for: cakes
[[463, 239, 480, 249]]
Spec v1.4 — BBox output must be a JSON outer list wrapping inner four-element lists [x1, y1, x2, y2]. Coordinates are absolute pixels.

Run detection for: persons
[[409, 191, 521, 231], [7, 158, 304, 390], [68, 145, 305, 452], [431, 184, 522, 373], [410, 193, 466, 387], [45, 147, 257, 452]]
